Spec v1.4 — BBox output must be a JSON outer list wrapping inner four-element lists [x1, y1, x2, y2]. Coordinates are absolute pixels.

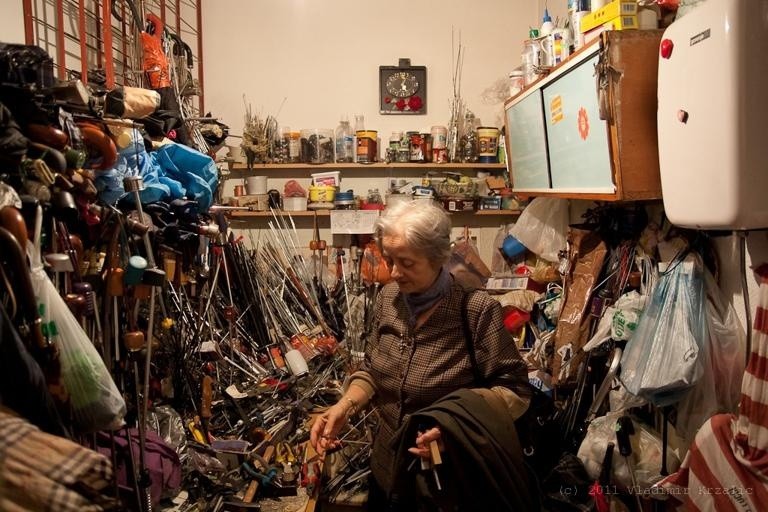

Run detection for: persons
[[310, 194, 532, 511]]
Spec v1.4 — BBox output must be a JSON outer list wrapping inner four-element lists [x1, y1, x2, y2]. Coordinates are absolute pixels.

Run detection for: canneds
[[283, 132, 301, 163]]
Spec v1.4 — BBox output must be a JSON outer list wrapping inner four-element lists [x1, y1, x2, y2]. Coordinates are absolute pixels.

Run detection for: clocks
[[380, 59, 427, 115]]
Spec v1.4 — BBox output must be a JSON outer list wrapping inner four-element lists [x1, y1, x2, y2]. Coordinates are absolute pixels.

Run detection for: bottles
[[508, 0, 658, 97], [272, 115, 505, 163]]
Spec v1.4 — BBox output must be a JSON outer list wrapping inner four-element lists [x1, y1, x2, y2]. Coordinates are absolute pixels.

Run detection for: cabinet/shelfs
[[215, 163, 523, 217], [504, 32, 663, 201]]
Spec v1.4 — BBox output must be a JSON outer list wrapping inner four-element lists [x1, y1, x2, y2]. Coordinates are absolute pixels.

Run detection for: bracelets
[[347, 398, 357, 413]]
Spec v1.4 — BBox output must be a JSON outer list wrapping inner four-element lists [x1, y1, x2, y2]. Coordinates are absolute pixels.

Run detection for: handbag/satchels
[[513, 381, 553, 462]]
